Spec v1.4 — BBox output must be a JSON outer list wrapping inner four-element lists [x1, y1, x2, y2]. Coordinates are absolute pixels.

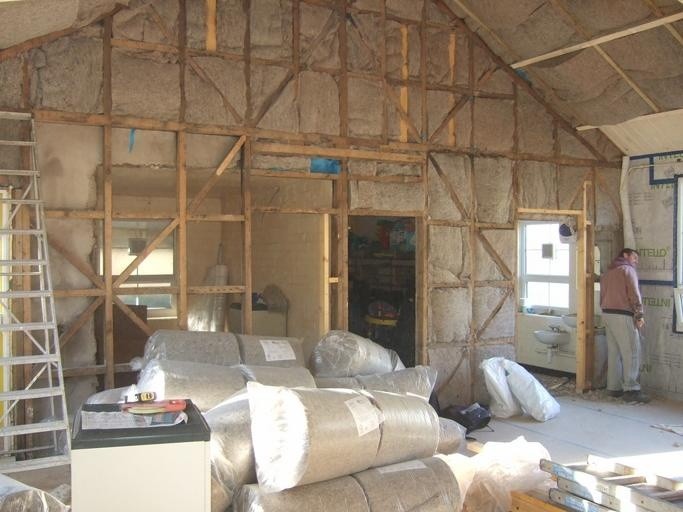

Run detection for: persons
[[599, 248, 652, 404]]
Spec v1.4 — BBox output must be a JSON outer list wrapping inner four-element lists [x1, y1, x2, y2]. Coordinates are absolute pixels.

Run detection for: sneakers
[[623, 390, 650, 403], [607, 390, 623, 397]]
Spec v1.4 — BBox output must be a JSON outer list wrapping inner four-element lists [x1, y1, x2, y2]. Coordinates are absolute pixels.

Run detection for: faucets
[[549, 325, 560, 332]]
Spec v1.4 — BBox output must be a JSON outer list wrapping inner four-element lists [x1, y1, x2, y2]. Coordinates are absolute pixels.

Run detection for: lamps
[[126, 235, 147, 304]]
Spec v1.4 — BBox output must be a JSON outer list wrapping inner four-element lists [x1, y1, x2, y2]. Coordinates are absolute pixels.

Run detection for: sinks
[[533, 329, 570, 346]]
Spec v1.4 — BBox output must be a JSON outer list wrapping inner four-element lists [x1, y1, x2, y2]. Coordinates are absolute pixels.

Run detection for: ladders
[[540, 454, 683, 512], [0, 111, 72, 475]]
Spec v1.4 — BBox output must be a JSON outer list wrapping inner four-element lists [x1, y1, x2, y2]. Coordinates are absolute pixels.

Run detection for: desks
[[69, 399, 211, 512], [230, 302, 286, 340], [96, 304, 147, 391], [511, 489, 571, 512]]
